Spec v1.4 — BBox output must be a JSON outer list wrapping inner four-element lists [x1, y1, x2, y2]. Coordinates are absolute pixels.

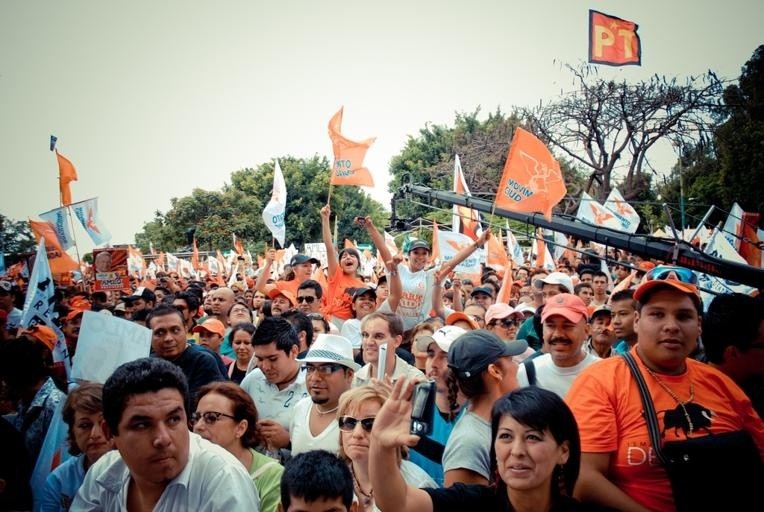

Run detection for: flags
[[260, 159, 290, 250], [583, 7, 643, 69], [49, 145, 77, 206], [496, 128, 568, 224], [452, 155, 486, 246], [327, 109, 377, 189], [573, 186, 641, 235]]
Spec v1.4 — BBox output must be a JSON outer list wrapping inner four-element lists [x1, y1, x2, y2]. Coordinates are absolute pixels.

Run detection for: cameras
[[409, 378, 437, 435]]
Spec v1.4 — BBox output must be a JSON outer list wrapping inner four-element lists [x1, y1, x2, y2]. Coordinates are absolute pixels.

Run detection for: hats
[[122, 287, 154, 302], [633, 264, 703, 305], [60, 307, 82, 320], [193, 319, 225, 337], [296, 334, 362, 373], [415, 272, 610, 377], [22, 324, 58, 350], [0, 281, 13, 293]]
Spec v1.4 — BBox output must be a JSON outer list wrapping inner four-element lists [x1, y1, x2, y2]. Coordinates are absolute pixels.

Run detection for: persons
[[0, 204, 764, 511]]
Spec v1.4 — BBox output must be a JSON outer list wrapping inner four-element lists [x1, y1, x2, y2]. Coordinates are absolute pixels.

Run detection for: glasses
[[302, 365, 342, 374], [352, 289, 377, 302], [643, 268, 697, 284], [269, 289, 297, 308], [297, 296, 317, 303], [291, 254, 319, 265], [338, 416, 375, 432], [190, 412, 233, 423], [409, 240, 431, 253]]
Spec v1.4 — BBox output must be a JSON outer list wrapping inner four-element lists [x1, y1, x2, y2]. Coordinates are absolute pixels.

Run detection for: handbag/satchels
[[663, 431, 764, 510]]
